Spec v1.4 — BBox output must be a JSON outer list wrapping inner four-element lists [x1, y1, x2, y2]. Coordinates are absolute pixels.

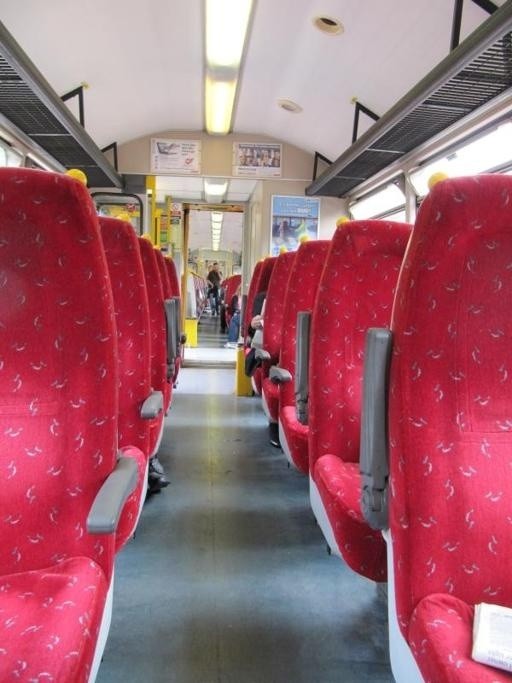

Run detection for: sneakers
[[212, 313, 220, 315], [149, 471, 170, 493], [224, 342, 237, 349], [220, 330, 225, 333]]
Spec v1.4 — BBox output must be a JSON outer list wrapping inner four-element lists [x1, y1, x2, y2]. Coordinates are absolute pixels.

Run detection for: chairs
[[3, 165, 147, 682], [359, 172, 512, 682], [295, 219, 417, 583], [135, 231, 333, 477], [96, 212, 166, 458]]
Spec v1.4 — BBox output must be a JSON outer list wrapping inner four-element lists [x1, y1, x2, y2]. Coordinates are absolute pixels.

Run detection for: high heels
[[269, 423, 280, 448]]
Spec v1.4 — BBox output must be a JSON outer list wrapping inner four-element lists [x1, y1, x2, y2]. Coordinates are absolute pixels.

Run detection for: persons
[[218, 284, 228, 332], [238, 147, 281, 167], [207, 265, 213, 276], [223, 284, 241, 348], [293, 218, 307, 241], [276, 219, 292, 244], [246, 290, 280, 447], [206, 263, 222, 315]]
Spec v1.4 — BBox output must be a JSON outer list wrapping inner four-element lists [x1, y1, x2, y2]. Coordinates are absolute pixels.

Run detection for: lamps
[[204, 0, 257, 137]]
